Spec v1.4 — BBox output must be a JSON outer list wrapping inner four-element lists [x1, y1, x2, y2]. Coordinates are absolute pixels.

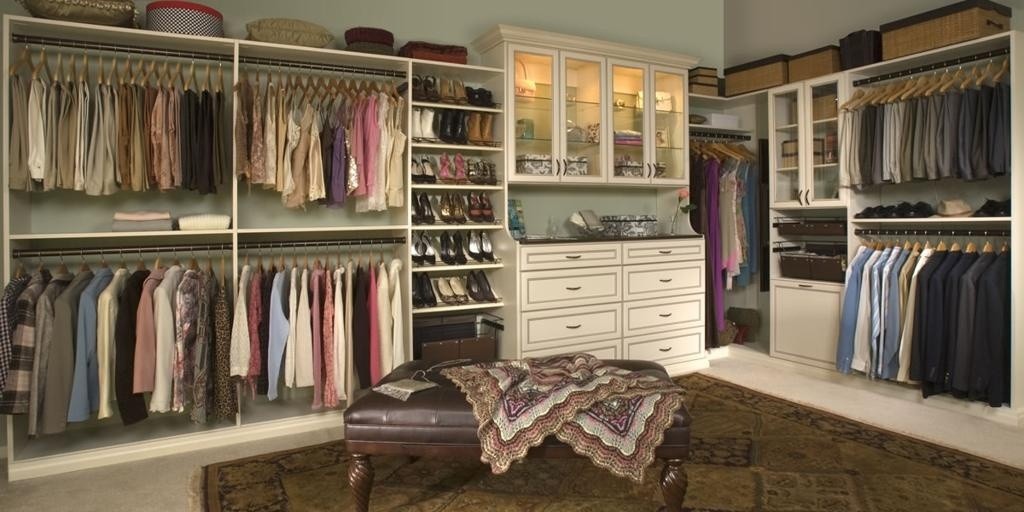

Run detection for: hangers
[[859, 228, 1009, 256], [689, 131, 758, 163], [840, 49, 1010, 111]]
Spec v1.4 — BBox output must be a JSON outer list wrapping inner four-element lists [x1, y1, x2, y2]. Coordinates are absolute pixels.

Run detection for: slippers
[[479, 88, 500, 107], [467, 86, 483, 106]]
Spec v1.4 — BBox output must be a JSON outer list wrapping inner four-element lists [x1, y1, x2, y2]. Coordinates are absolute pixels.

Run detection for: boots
[[412, 106, 422, 143], [467, 113, 483, 144], [454, 111, 466, 144], [441, 110, 453, 143], [481, 114, 493, 145], [420, 108, 440, 142]]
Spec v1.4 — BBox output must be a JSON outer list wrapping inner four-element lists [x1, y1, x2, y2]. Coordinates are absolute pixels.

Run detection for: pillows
[[244, 17, 334, 48], [19, 0, 139, 29]]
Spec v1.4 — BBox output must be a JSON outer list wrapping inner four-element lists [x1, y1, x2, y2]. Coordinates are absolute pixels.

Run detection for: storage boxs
[[147, 0, 223, 39], [516, 152, 666, 238], [879, 0, 1012, 61], [724, 29, 882, 98]]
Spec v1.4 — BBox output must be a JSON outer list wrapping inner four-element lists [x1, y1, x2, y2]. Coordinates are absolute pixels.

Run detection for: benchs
[[343, 359, 692, 512]]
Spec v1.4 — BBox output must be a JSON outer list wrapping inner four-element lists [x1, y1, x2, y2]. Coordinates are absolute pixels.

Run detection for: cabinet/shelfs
[[3, 15, 505, 482], [482, 23, 768, 367], [769, 32, 1024, 427]]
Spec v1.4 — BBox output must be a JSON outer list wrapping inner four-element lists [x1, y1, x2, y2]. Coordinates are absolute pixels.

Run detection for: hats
[[929, 199, 973, 218]]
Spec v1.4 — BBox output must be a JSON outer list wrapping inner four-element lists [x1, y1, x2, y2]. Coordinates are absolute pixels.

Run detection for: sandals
[[412, 73, 427, 101], [424, 75, 440, 101]]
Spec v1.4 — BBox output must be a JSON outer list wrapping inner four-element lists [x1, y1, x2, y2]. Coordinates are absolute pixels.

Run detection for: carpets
[[188, 371, 1023, 511]]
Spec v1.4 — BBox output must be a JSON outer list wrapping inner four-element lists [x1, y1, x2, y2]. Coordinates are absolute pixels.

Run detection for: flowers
[[670, 189, 697, 233]]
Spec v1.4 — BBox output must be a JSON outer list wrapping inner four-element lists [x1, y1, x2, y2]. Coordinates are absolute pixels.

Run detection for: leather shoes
[[855, 206, 882, 218], [437, 277, 457, 305], [866, 206, 894, 218], [449, 276, 468, 304], [974, 200, 999, 216], [412, 273, 424, 308], [890, 202, 912, 217], [420, 273, 437, 306], [905, 201, 936, 218], [999, 199, 1011, 216]]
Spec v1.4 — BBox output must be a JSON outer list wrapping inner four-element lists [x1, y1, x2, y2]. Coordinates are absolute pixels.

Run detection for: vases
[[670, 215, 677, 237]]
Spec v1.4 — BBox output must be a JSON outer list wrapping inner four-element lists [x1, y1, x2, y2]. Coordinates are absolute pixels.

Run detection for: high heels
[[452, 192, 466, 224], [420, 156, 436, 183], [412, 231, 424, 265], [467, 159, 485, 184], [453, 231, 466, 264], [441, 231, 456, 265], [467, 229, 483, 261], [420, 193, 435, 224], [477, 271, 495, 302], [454, 74, 469, 105], [440, 151, 454, 184], [466, 271, 484, 301], [441, 192, 454, 221], [482, 161, 497, 184], [480, 192, 495, 221], [422, 231, 436, 265], [412, 153, 424, 182], [412, 190, 423, 224], [454, 153, 466, 184], [468, 192, 484, 221], [479, 230, 494, 262], [440, 72, 456, 104]]
